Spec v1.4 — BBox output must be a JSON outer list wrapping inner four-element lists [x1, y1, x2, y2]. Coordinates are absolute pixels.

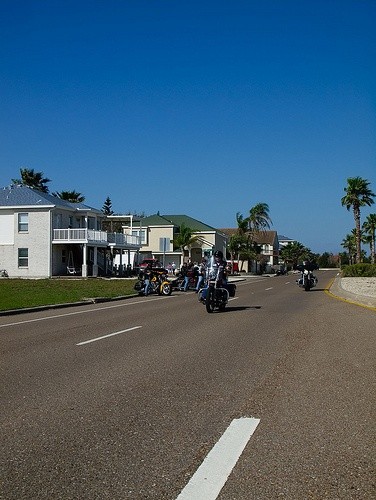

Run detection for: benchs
[[66, 266, 82, 275]]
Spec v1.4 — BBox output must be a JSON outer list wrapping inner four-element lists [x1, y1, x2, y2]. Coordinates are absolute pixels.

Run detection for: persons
[[179, 260, 205, 293], [114, 263, 133, 278], [168, 262, 176, 275], [198, 251, 231, 303], [144, 264, 153, 296], [298, 261, 318, 285]]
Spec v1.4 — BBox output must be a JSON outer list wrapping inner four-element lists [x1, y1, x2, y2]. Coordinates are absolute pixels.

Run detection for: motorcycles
[[131, 268, 207, 296], [296, 261, 319, 291], [198, 255, 229, 313]]
